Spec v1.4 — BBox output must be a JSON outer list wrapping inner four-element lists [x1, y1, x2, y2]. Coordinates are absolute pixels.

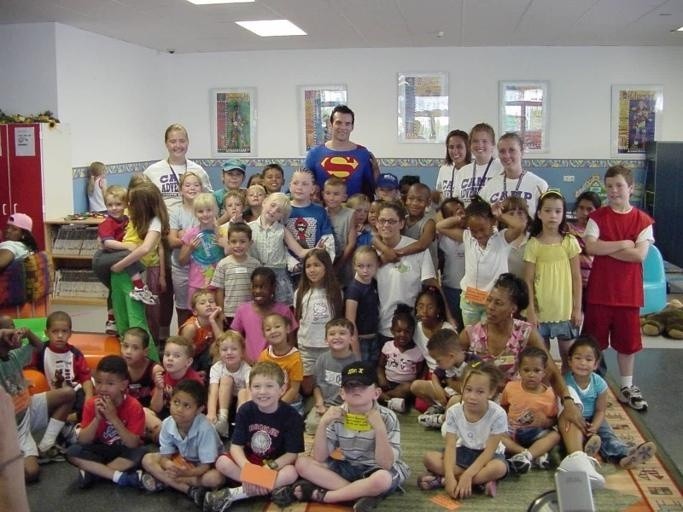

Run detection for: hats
[[9, 213, 32, 230], [375, 173, 400, 189], [341, 361, 378, 388], [220, 158, 246, 173]]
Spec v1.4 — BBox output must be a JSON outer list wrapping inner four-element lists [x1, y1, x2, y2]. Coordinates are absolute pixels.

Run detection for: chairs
[[0, 248, 52, 317], [636, 242, 667, 316]]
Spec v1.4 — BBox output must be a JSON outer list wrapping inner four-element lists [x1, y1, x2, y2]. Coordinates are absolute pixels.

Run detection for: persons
[[146, 124, 212, 356], [451, 123, 503, 205], [632, 101, 648, 147], [303, 105, 380, 199], [475, 132, 547, 218], [1, 160, 660, 512], [434, 130, 471, 200]]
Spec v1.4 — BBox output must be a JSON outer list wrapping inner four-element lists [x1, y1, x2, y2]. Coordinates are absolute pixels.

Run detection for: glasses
[[377, 218, 396, 224]]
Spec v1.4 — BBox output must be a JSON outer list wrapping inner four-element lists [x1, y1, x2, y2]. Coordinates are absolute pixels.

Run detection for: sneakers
[[385, 384, 657, 498], [130, 283, 161, 306], [271, 485, 295, 507], [37, 420, 232, 512]]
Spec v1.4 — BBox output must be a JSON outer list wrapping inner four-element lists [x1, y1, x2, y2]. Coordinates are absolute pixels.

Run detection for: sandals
[[353, 496, 383, 512], [292, 480, 329, 504]]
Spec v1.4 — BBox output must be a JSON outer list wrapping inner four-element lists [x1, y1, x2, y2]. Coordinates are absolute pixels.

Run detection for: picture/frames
[[496, 80, 550, 154], [610, 82, 667, 161], [396, 71, 450, 144], [206, 84, 261, 159], [297, 82, 354, 158]]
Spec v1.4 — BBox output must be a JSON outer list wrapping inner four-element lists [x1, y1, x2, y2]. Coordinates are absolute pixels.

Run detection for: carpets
[[0, 328, 683, 510]]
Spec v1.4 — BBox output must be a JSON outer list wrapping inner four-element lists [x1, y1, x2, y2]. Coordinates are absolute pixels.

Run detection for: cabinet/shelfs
[[0, 123, 75, 283], [44, 214, 128, 334], [640, 140, 682, 267]]
